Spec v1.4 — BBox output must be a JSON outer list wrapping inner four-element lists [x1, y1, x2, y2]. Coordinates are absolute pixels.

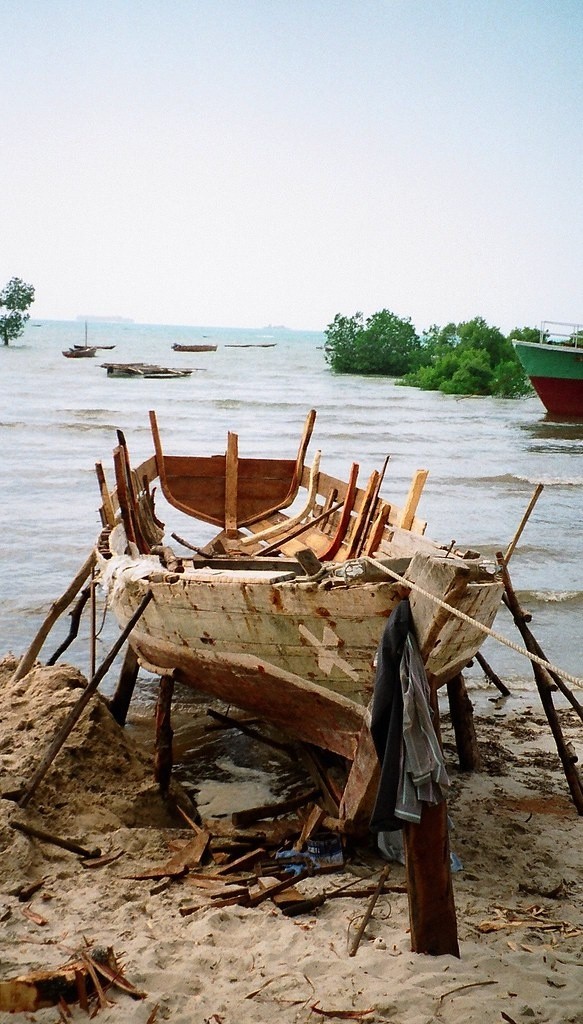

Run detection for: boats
[[62, 321, 116, 358], [512, 320, 583, 417], [171, 343, 218, 352], [95, 410, 504, 766]]
